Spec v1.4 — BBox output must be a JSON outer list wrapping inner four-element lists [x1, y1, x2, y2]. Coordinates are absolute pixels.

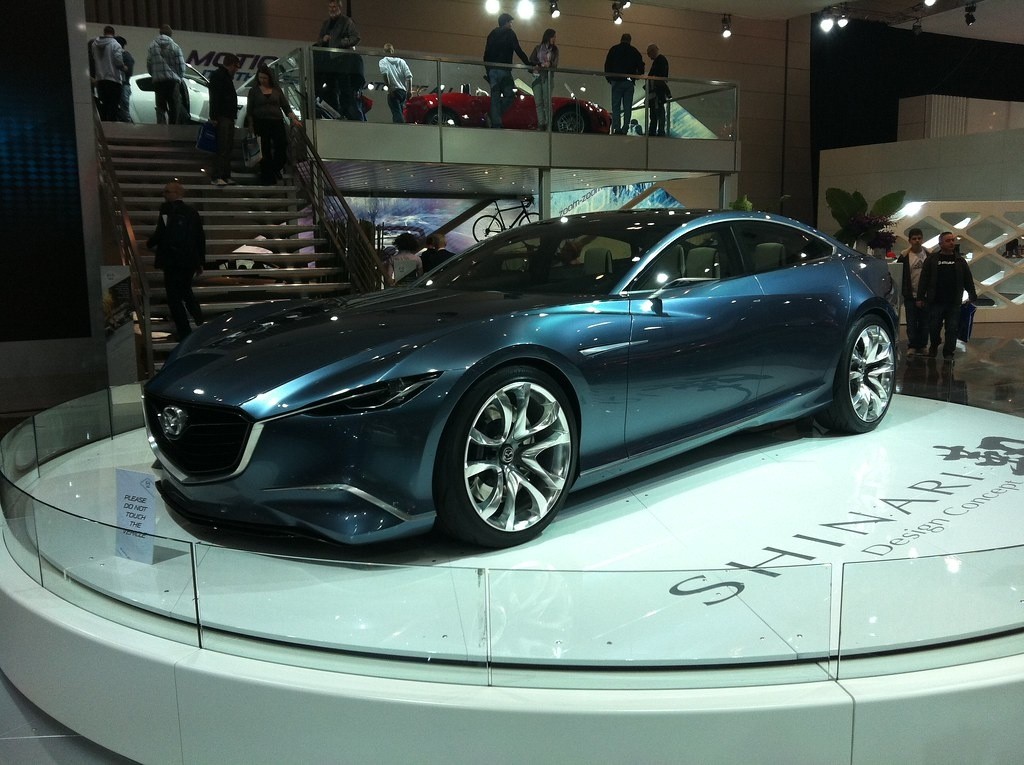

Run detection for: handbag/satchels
[[957, 302, 977, 343], [241, 132, 263, 167], [196, 124, 216, 153]]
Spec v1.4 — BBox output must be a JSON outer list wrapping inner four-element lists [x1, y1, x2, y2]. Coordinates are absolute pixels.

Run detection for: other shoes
[[211, 179, 227, 185], [929, 342, 941, 357], [908, 348, 916, 355], [222, 178, 236, 184], [942, 345, 955, 359], [167, 334, 184, 342]]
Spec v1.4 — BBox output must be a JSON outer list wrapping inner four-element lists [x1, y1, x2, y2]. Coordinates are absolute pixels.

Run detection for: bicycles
[[472, 197, 539, 246]]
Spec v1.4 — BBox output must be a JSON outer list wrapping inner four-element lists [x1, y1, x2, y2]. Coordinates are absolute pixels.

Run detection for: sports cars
[[94, 63, 301, 128], [140, 208, 902, 551], [403, 78, 612, 133]]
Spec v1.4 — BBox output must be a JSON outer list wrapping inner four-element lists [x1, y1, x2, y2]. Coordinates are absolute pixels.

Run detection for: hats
[[160, 25, 173, 36]]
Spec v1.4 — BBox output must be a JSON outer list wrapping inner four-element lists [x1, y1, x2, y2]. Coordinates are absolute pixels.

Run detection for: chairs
[[460, 83, 471, 94], [583, 248, 613, 275], [685, 248, 723, 278], [755, 243, 786, 273]]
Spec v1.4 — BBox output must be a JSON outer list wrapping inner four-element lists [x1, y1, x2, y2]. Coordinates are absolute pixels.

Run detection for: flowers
[[868, 230, 898, 252], [846, 212, 901, 240]]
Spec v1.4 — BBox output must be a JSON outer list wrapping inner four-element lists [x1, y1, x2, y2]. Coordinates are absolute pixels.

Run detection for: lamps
[[611, 0, 631, 25], [721, 17, 732, 38], [549, 0, 560, 19], [964, 6, 976, 26], [912, 20, 923, 35]]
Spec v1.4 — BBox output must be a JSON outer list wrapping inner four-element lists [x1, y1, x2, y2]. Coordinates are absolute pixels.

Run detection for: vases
[[875, 248, 886, 258], [856, 239, 867, 254]]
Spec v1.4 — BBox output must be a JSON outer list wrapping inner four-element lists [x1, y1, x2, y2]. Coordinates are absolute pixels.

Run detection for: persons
[[897, 228, 932, 354], [209, 54, 243, 186], [483, 14, 530, 128], [387, 233, 424, 284], [627, 119, 638, 135], [529, 29, 560, 132], [87, 26, 135, 122], [642, 44, 673, 137], [136, 183, 206, 342], [246, 67, 303, 184], [421, 233, 457, 274], [604, 33, 645, 135], [316, 1, 368, 122], [378, 43, 413, 124], [915, 232, 978, 362], [146, 25, 192, 124]]
